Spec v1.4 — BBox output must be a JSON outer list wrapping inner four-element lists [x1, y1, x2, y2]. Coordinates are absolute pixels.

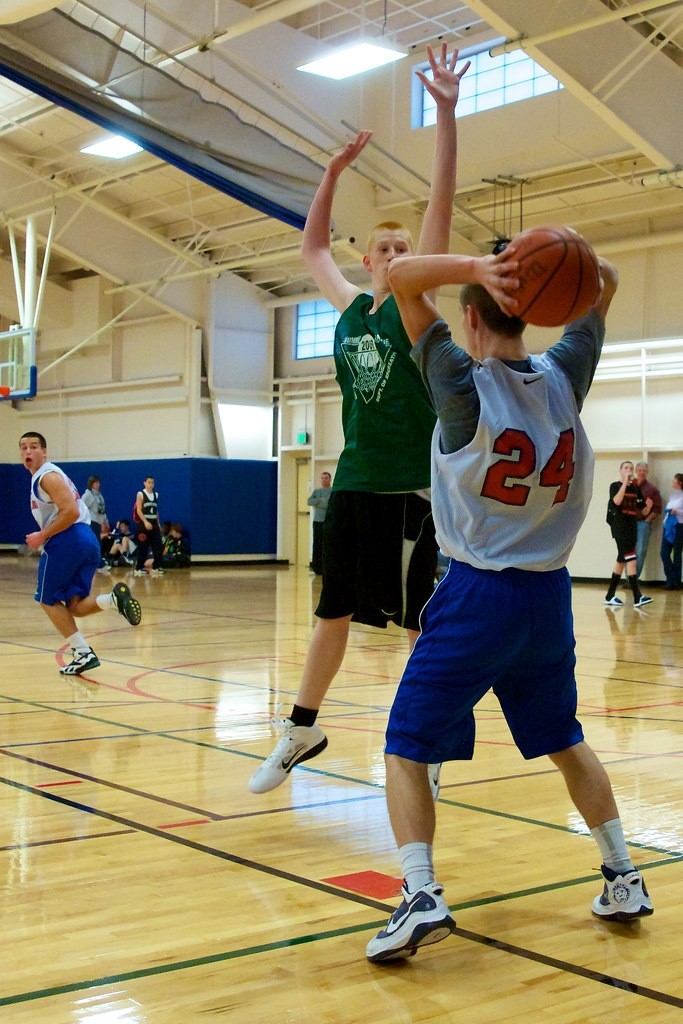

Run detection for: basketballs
[[501, 223, 602, 327]]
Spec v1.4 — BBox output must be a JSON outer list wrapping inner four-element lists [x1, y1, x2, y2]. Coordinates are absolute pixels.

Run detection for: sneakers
[[110, 582, 141, 626], [365, 878, 456, 961], [59, 646, 100, 675], [248, 703, 327, 794], [592, 861, 654, 921], [427, 762, 443, 803]]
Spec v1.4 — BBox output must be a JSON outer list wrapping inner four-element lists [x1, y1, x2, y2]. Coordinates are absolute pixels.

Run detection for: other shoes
[[152, 568, 168, 574], [97, 565, 112, 574], [120, 555, 134, 567], [633, 596, 653, 608], [603, 596, 623, 606], [134, 569, 149, 577]]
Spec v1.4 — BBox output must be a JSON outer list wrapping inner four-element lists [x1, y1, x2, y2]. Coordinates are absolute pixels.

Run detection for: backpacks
[[133, 499, 144, 524]]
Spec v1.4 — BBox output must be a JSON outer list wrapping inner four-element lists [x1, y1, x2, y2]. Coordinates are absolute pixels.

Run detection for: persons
[[307, 472, 334, 574], [81, 475, 111, 570], [365, 249, 654, 965], [602, 460, 653, 606], [623, 461, 663, 591], [248, 40, 472, 795], [134, 476, 168, 575], [19, 431, 142, 673], [102, 521, 191, 576], [661, 474, 683, 592]]
